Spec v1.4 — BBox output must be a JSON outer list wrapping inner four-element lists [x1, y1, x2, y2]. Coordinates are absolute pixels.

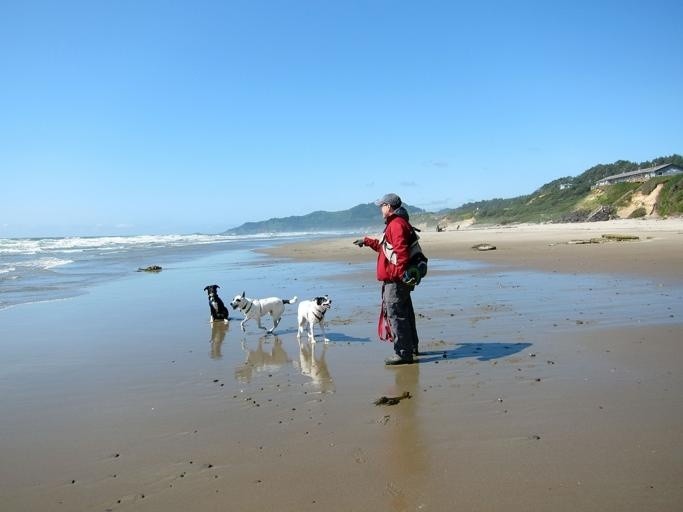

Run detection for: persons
[[353, 192, 419, 365]]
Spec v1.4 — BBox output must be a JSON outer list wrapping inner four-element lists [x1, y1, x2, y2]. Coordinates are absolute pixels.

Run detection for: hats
[[375, 193, 402, 208]]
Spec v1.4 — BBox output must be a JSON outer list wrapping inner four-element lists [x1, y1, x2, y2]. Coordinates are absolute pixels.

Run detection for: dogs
[[297, 295, 331, 344], [230, 291, 297, 334], [293, 344, 336, 395], [204, 285, 231, 323], [232, 334, 293, 383], [209, 323, 230, 359]]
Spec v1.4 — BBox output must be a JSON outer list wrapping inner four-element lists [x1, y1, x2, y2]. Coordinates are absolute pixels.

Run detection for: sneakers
[[384, 349, 418, 365]]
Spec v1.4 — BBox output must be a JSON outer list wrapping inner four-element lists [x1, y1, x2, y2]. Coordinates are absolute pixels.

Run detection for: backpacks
[[407, 221, 428, 291]]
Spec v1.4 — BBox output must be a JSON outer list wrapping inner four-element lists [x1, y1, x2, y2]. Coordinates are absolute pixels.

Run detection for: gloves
[[353, 238, 364, 248], [400, 270, 417, 286]]
